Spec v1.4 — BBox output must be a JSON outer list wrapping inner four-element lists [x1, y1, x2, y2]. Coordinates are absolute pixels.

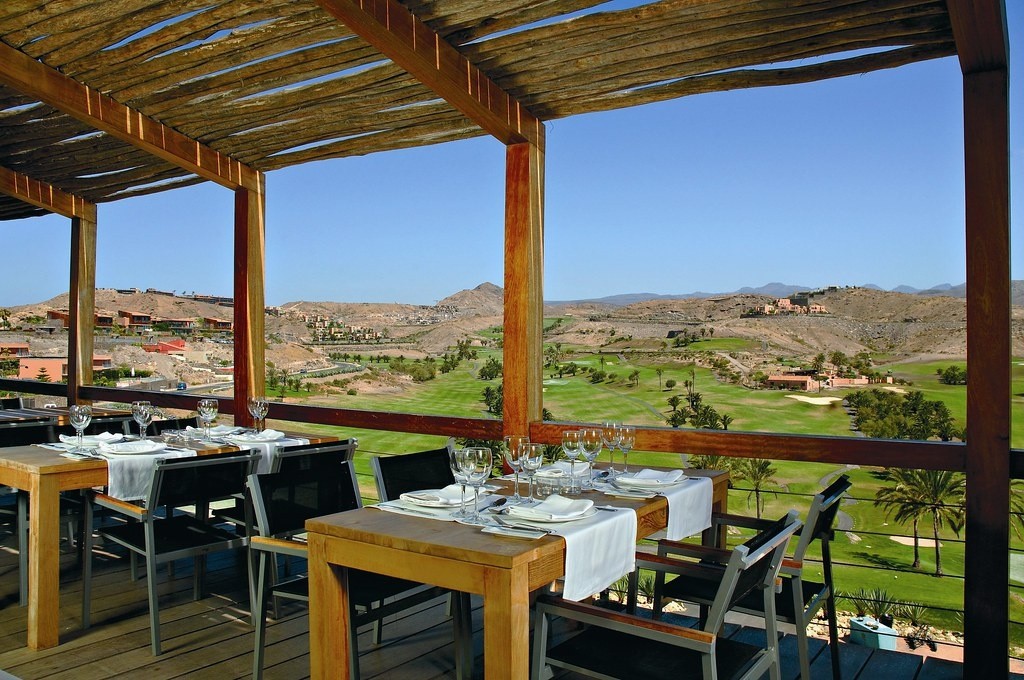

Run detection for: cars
[[300, 369, 307, 373], [217, 339, 234, 344]]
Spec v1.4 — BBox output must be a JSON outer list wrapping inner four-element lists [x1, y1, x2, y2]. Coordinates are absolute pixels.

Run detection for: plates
[[480, 526, 548, 540], [538, 473, 589, 479], [82, 435, 99, 446], [612, 472, 690, 489], [399, 488, 488, 508], [503, 505, 598, 523], [112, 451, 152, 455], [604, 492, 658, 498]]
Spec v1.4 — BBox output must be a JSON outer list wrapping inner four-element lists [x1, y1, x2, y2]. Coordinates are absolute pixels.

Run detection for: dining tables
[[304, 460, 730, 680], [0, 426, 341, 653], [0, 405, 153, 549]]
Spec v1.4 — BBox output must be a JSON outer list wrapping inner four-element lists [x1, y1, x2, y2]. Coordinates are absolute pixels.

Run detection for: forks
[[490, 515, 552, 535], [608, 481, 664, 495]]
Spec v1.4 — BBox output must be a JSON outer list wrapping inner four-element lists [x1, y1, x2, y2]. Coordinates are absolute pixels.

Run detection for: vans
[[176, 382, 186, 390]]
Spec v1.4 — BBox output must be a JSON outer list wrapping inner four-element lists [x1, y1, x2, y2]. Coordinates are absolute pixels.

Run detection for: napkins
[[509, 494, 594, 520], [229, 429, 285, 441], [400, 484, 482, 506], [614, 469, 684, 484], [535, 461, 589, 477], [186, 425, 240, 435], [58, 431, 123, 446], [100, 440, 168, 453]]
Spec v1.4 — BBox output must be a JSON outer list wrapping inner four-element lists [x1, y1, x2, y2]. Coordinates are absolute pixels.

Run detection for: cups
[[536, 472, 592, 496], [159, 429, 194, 449]]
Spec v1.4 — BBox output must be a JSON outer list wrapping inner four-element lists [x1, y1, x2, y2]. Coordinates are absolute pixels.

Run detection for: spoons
[[586, 471, 609, 483], [475, 498, 507, 513]]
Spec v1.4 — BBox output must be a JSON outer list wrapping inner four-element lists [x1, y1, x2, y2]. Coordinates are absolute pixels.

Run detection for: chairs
[[246, 461, 466, 680], [0, 397, 24, 411], [652, 473, 852, 679], [0, 421, 104, 606], [80, 447, 263, 656], [103, 416, 218, 582], [211, 438, 360, 618], [368, 444, 460, 646], [531, 510, 803, 680]]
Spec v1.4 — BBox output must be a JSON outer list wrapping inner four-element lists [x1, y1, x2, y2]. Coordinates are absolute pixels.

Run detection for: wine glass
[[503, 435, 530, 505], [246, 395, 269, 435], [461, 446, 492, 523], [448, 449, 478, 518], [68, 404, 93, 453], [197, 398, 219, 443], [613, 425, 636, 475], [601, 420, 623, 476], [579, 427, 604, 490], [131, 400, 155, 444], [561, 430, 587, 495], [517, 442, 544, 504]]
